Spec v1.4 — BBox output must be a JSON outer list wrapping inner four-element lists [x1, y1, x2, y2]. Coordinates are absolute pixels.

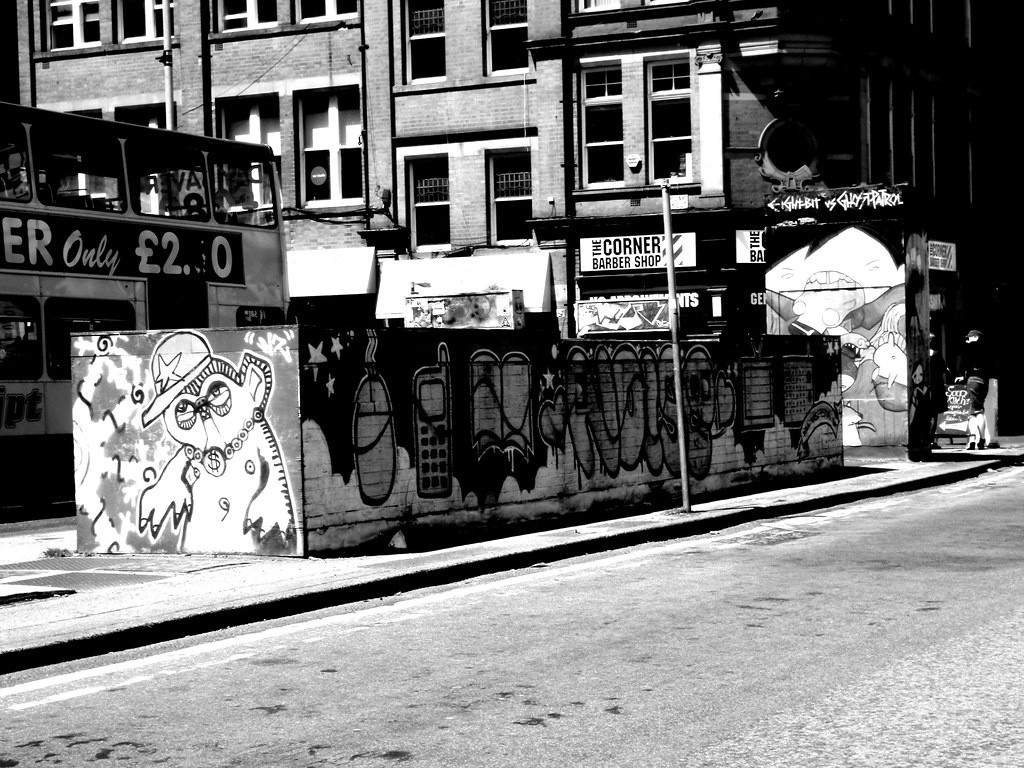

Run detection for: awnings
[[376, 254, 552, 320], [287, 246, 376, 296]]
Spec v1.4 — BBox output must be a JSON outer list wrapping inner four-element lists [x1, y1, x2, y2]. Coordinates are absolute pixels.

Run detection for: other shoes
[[967, 442, 975, 450], [977, 438, 986, 449]]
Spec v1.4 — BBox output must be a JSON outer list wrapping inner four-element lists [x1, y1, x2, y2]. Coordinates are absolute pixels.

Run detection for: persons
[[930, 332, 949, 449], [956, 330, 989, 450]]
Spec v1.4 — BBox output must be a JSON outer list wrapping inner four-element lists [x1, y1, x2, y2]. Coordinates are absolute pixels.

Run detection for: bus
[[1, 102, 290, 508]]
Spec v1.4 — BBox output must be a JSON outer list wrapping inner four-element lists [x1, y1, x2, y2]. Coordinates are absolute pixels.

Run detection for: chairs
[[31, 183, 111, 212]]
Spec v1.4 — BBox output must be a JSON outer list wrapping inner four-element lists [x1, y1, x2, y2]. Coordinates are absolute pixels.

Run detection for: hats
[[965, 330, 982, 337]]
